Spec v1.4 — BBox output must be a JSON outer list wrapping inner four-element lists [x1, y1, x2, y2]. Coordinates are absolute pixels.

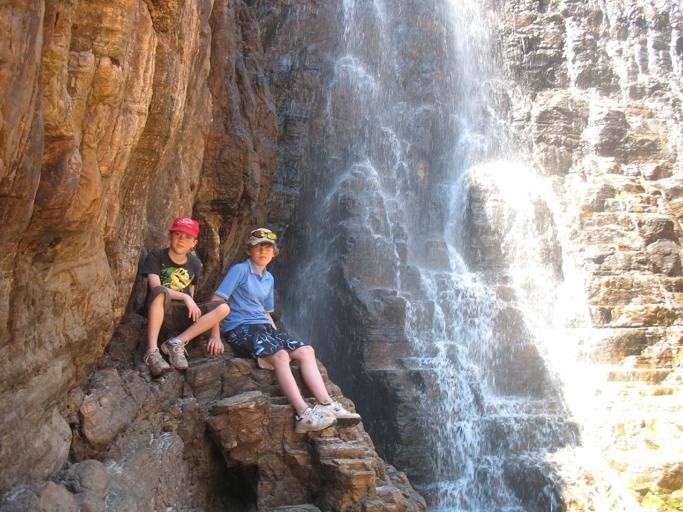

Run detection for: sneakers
[[144, 348, 171, 377], [160, 337, 189, 369], [295, 401, 361, 434]]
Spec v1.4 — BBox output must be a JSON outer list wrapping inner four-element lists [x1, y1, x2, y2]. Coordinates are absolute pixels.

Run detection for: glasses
[[248, 231, 277, 240]]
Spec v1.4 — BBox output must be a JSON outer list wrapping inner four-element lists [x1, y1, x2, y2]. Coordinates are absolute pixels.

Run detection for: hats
[[168, 217, 200, 237], [246, 227, 276, 246]]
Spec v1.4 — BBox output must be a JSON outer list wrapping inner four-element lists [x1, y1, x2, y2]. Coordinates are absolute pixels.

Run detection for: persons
[[135, 216, 230, 378], [202, 227, 362, 435]]
